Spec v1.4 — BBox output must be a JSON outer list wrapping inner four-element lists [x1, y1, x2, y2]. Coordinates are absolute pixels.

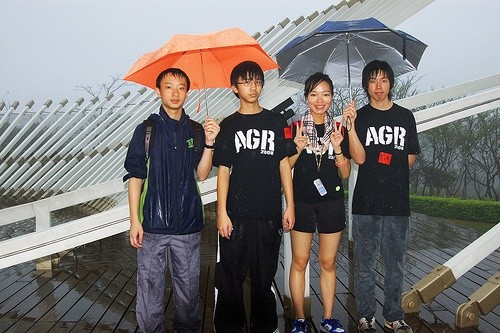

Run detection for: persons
[[123, 67, 220, 333], [285, 73, 352, 333], [343, 59, 421, 333], [213, 60, 296, 333]]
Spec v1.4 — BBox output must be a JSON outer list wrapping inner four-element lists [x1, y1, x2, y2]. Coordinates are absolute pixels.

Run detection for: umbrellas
[[120, 26, 282, 119], [276, 17, 429, 120]]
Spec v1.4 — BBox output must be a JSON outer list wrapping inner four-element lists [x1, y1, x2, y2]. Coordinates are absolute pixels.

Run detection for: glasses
[[237, 81, 263, 87]]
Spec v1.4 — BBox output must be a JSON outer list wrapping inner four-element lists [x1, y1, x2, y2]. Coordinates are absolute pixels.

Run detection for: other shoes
[[273, 328, 280, 333]]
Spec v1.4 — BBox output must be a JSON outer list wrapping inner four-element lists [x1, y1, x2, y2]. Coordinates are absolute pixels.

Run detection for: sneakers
[[320, 316, 346, 333], [358, 314, 376, 333], [290, 318, 308, 333], [384, 318, 413, 333]]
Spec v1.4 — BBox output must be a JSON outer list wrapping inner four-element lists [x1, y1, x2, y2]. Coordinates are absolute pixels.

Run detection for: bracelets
[[334, 151, 343, 155], [204, 143, 216, 149], [334, 156, 349, 167]]
